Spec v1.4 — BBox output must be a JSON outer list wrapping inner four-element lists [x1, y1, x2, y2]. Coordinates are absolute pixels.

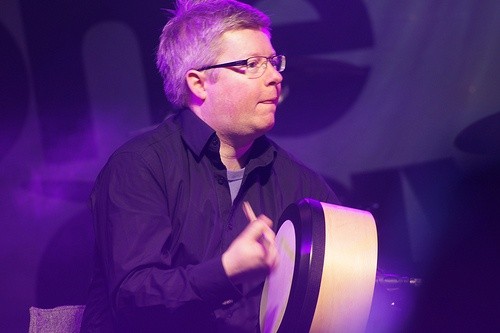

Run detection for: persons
[[78, 0, 345, 333]]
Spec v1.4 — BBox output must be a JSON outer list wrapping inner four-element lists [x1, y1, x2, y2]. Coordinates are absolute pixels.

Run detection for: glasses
[[196, 54, 286, 78]]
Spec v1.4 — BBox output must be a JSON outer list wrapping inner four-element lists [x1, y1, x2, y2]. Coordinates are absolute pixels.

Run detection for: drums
[[259, 198, 379, 333]]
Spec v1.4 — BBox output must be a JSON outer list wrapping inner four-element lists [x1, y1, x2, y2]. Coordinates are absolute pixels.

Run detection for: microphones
[[375, 270, 429, 290]]
